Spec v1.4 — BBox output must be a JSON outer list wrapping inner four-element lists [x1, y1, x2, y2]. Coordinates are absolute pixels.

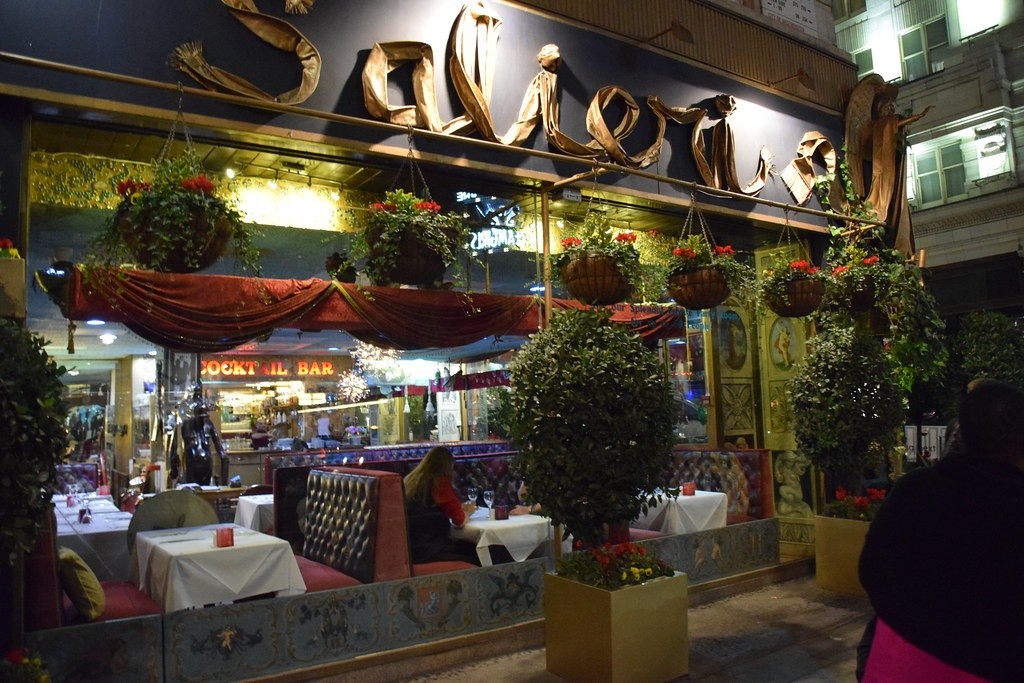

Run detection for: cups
[[467, 488, 479, 500]]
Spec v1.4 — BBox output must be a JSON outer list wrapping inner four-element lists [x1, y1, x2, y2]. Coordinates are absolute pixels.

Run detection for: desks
[[132, 523, 308, 615], [195, 485, 274, 532], [631, 486, 729, 535], [448, 505, 555, 566], [50, 492, 134, 580]]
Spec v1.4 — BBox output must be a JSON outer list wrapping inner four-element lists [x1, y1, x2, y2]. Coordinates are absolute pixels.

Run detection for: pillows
[[55, 545, 106, 622]]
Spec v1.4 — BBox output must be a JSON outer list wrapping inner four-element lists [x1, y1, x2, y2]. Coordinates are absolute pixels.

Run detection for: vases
[[845, 275, 883, 310], [123, 213, 232, 273], [560, 255, 639, 307], [665, 265, 734, 309], [365, 223, 462, 283], [545, 570, 690, 682], [814, 514, 874, 602], [767, 278, 826, 317]]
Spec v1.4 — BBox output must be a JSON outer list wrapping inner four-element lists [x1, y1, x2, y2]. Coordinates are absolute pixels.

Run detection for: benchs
[[26, 509, 164, 634], [263, 440, 774, 597]]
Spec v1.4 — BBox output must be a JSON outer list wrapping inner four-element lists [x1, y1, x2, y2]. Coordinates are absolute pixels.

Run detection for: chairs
[[244, 484, 273, 496], [54, 464, 98, 495]]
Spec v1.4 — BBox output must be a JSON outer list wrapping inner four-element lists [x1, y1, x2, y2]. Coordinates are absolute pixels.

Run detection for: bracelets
[[528, 504, 532, 514]]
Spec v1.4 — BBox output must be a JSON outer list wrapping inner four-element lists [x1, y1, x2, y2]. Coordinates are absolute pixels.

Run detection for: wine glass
[[483, 490, 495, 518], [64, 484, 95, 527]]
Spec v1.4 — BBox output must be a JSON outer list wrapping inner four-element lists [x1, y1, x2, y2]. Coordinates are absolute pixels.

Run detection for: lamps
[[33, 260, 75, 292], [767, 68, 817, 92], [644, 22, 696, 45], [911, 248, 927, 269], [326, 251, 358, 284]]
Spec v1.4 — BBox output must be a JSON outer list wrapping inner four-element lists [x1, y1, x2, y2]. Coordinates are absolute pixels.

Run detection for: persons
[[856, 379, 1024, 683], [725, 436, 748, 448], [510, 437, 546, 516], [776, 450, 811, 516], [67, 407, 102, 447], [677, 406, 708, 442], [254, 417, 269, 434], [56, 474, 92, 493], [404, 446, 477, 568]]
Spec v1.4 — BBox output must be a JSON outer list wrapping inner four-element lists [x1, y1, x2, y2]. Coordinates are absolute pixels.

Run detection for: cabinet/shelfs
[[224, 450, 267, 489]]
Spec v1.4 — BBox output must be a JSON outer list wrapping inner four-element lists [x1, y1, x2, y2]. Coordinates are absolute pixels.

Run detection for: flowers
[[0, 648, 51, 683], [558, 539, 674, 588], [822, 486, 888, 521], [328, 188, 487, 305], [529, 209, 898, 309], [81, 146, 272, 305]]
